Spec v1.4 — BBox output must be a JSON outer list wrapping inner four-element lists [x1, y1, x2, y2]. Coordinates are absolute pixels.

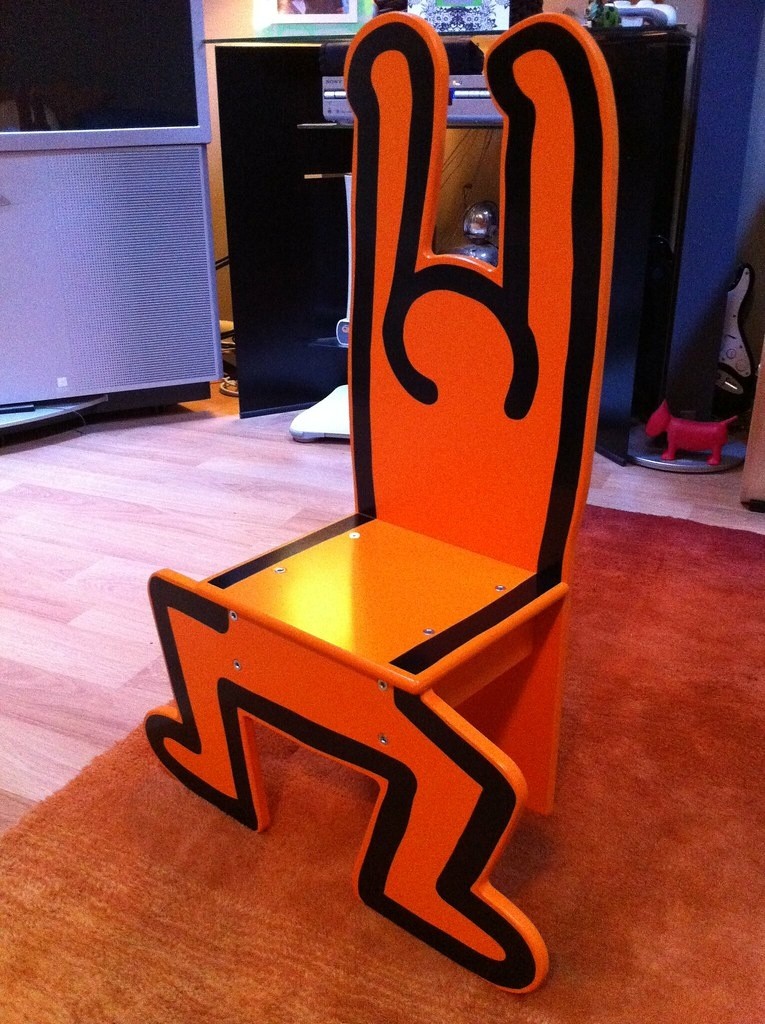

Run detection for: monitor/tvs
[[0, 0, 211, 152]]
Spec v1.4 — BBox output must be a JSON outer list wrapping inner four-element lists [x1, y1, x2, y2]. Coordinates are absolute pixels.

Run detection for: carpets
[[2, 505, 765, 1024]]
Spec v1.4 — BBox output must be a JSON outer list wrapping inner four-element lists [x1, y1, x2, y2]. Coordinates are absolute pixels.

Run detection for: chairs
[[144, 11, 620, 991]]
